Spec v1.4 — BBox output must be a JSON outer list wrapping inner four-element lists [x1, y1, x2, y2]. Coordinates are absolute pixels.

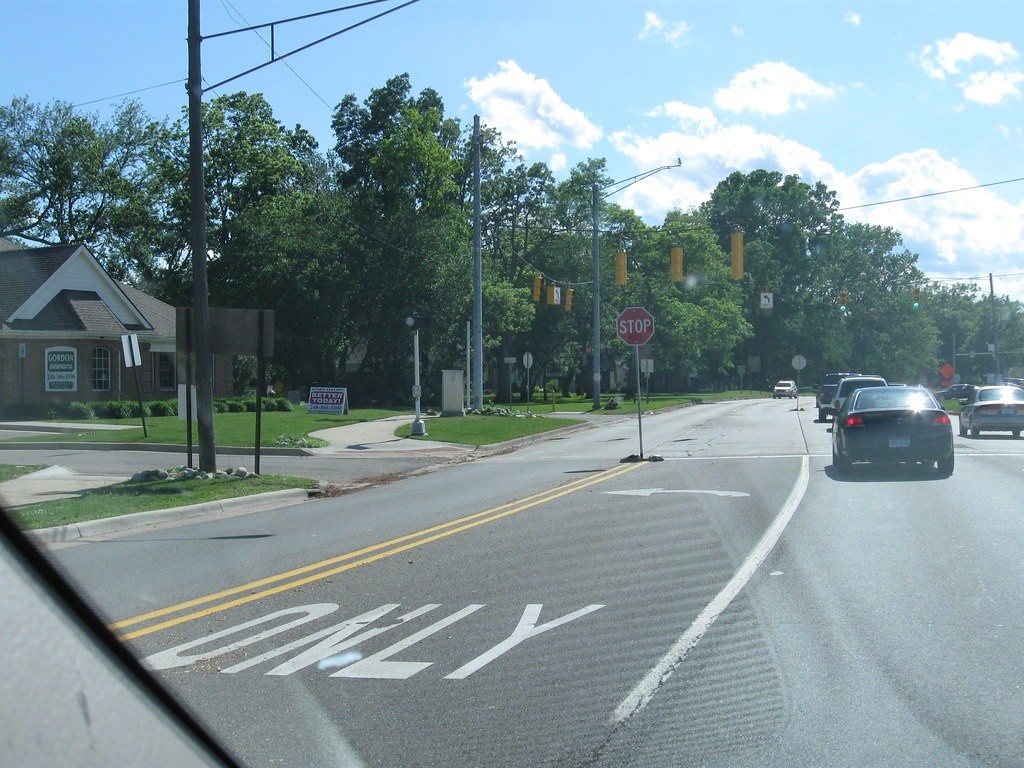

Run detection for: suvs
[[772, 379, 798, 399]]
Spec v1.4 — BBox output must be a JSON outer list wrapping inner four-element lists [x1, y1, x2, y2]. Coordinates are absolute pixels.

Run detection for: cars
[[829, 374, 889, 423], [934, 383, 976, 401], [818, 385, 954, 478], [957, 385, 1024, 439], [813, 373, 863, 422], [888, 382, 906, 386], [1003, 377, 1024, 389]]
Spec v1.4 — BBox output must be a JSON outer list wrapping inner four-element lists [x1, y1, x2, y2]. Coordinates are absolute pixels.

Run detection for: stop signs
[[615, 307, 654, 346]]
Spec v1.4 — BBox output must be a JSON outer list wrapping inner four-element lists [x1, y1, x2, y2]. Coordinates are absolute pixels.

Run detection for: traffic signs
[[761, 293, 773, 308]]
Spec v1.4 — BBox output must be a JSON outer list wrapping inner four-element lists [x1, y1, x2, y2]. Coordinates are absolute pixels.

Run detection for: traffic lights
[[840, 292, 847, 311], [404, 316, 417, 329], [913, 288, 920, 309]]
